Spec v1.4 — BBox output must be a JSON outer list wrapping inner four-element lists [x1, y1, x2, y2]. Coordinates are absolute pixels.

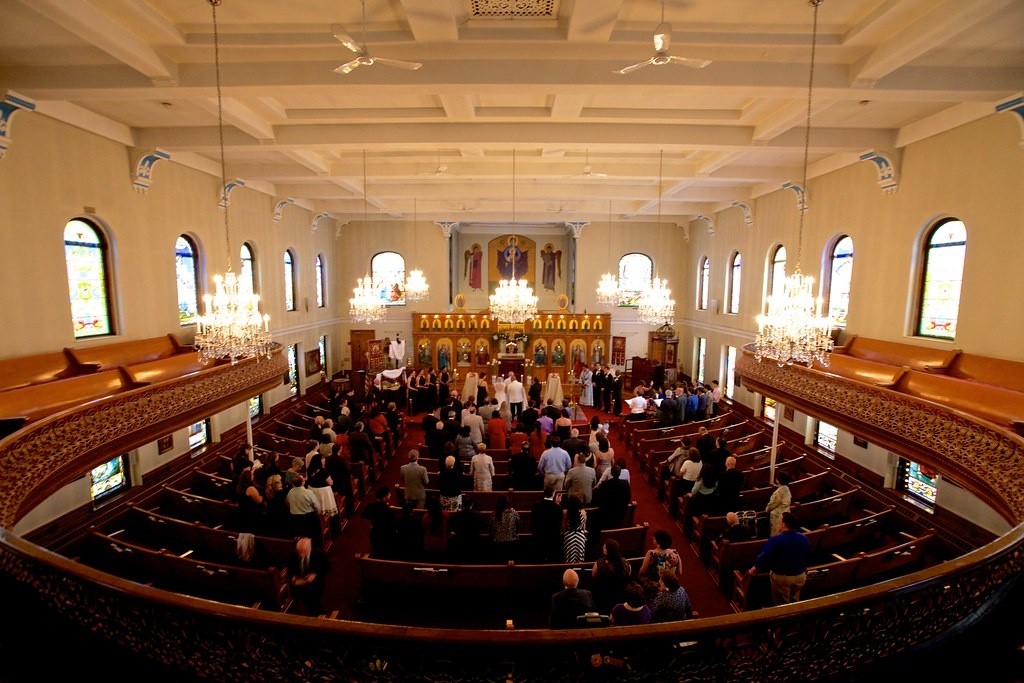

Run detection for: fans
[[331, 0, 423, 75], [565, 149, 606, 179], [610, 0, 714, 76], [423, 152, 453, 177]]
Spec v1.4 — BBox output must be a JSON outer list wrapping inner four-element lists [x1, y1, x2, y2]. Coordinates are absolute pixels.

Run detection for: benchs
[[0, 331, 238, 441], [85, 391, 939, 620], [812, 334, 1024, 435]]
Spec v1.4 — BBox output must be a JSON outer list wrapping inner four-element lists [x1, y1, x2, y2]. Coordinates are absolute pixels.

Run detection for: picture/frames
[[304, 347, 322, 378], [853, 435, 867, 449], [733, 370, 740, 387], [783, 405, 794, 421], [157, 434, 174, 455]]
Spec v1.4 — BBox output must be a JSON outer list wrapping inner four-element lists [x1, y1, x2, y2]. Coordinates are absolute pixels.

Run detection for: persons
[[231, 441, 252, 485], [362, 363, 631, 565], [307, 454, 338, 517], [714, 512, 753, 555], [660, 426, 745, 505], [592, 539, 631, 579], [400, 449, 430, 509], [288, 538, 330, 614], [238, 388, 403, 516], [755, 513, 813, 605], [548, 569, 592, 630], [418, 342, 488, 368], [765, 471, 792, 538], [638, 530, 682, 592], [650, 571, 691, 623], [535, 341, 603, 365], [610, 583, 650, 625], [285, 475, 321, 537], [232, 533, 268, 570], [622, 380, 721, 429]]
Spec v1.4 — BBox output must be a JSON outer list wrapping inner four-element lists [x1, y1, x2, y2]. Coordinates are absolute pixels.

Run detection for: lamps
[[348, 150, 388, 324], [488, 148, 539, 324], [595, 199, 624, 306], [193, 0, 273, 367], [754, 0, 839, 369], [637, 149, 676, 325], [403, 198, 431, 302]]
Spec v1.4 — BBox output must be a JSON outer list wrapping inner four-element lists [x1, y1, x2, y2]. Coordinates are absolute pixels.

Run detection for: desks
[[332, 378, 350, 391]]
